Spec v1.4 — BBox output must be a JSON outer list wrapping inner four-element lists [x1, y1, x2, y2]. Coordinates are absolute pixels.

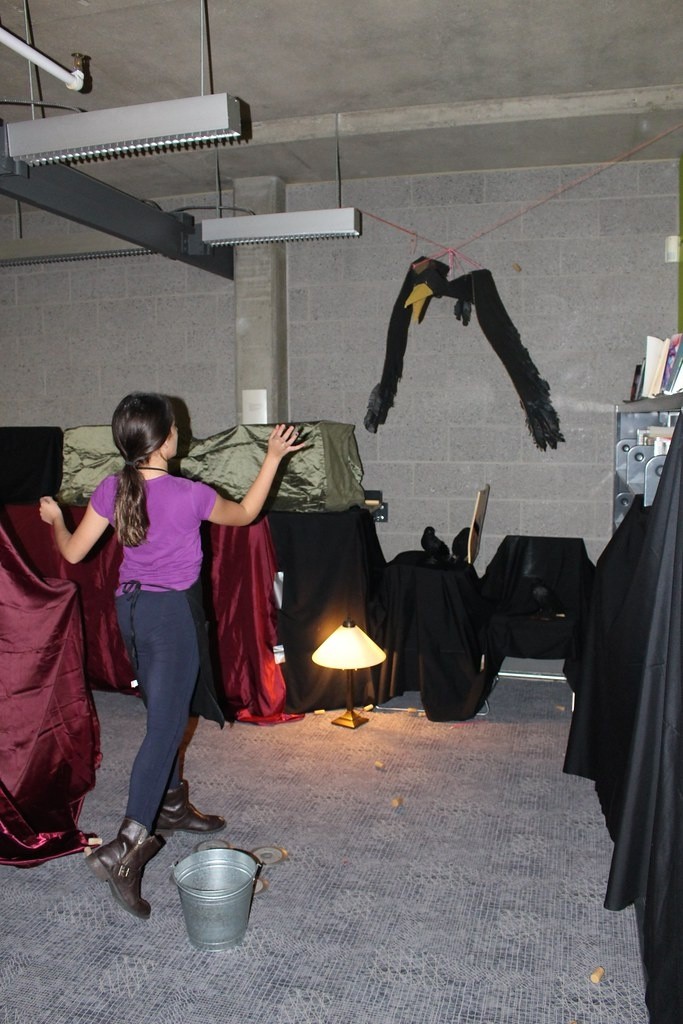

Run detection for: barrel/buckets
[[171, 847, 261, 952]]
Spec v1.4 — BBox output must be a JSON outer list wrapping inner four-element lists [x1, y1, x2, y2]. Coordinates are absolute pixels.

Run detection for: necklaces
[[136, 465, 169, 474]]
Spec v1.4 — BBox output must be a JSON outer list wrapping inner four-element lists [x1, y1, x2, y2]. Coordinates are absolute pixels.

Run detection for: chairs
[[466, 484, 491, 562]]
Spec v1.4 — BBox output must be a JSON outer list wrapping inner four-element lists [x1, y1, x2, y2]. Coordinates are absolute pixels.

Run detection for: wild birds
[[362, 255, 564, 452]]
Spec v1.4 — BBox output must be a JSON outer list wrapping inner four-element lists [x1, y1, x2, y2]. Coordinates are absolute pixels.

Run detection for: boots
[[86, 818, 162, 920], [154, 781, 232, 836]]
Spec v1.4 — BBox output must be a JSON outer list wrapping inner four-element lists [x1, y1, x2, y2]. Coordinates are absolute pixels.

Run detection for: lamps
[[311, 617, 387, 733], [7, 92, 241, 166], [202, 207, 362, 248]]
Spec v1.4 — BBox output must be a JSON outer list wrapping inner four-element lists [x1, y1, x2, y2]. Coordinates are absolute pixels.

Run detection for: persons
[[40, 393, 304, 918]]
